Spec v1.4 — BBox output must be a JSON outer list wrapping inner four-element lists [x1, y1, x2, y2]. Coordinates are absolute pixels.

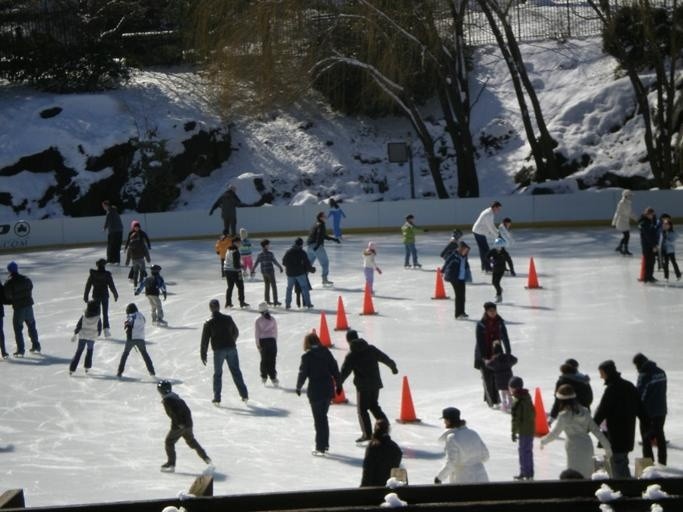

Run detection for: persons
[[254, 302, 280, 388], [594, 361, 651, 479], [483, 238, 516, 304], [360, 419, 390, 487], [565, 359, 591, 384], [653, 215, 666, 272], [102, 200, 118, 263], [639, 208, 661, 284], [157, 380, 211, 472], [215, 228, 233, 280], [540, 384, 613, 480], [441, 241, 472, 321], [493, 217, 516, 277], [440, 228, 463, 262], [117, 303, 157, 376], [339, 329, 398, 448], [201, 299, 248, 407], [328, 202, 346, 238], [611, 189, 639, 255], [483, 340, 518, 413], [363, 241, 381, 297], [308, 212, 342, 288], [70, 301, 102, 375], [295, 333, 343, 453], [84, 259, 119, 338], [251, 240, 284, 308], [656, 213, 683, 282], [0, 281, 10, 356], [282, 238, 316, 310], [209, 184, 241, 241], [634, 353, 667, 465], [134, 265, 168, 326], [110, 206, 123, 264], [367, 429, 403, 487], [223, 237, 251, 308], [234, 228, 256, 276], [295, 240, 318, 307], [547, 364, 594, 435], [508, 377, 535, 481], [127, 233, 151, 294], [125, 221, 151, 279], [400, 215, 429, 270], [472, 201, 503, 275], [474, 302, 512, 408], [434, 407, 489, 484], [3, 262, 40, 355]]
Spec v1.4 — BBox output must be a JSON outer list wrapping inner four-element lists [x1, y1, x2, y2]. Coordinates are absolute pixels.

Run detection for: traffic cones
[[525, 255, 544, 290], [333, 295, 350, 331], [430, 267, 452, 300], [395, 375, 422, 424], [329, 380, 347, 404], [525, 256, 542, 291], [638, 255, 645, 282], [636, 255, 648, 281], [431, 269, 450, 300], [396, 377, 419, 423], [334, 295, 352, 331], [331, 375, 350, 404], [311, 327, 316, 334]]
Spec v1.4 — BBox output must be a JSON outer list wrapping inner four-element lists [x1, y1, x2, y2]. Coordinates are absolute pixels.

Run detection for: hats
[[555, 384, 577, 399], [369, 241, 377, 250], [240, 229, 247, 238], [8, 260, 17, 271], [440, 408, 460, 419], [510, 378, 523, 387], [210, 300, 219, 312], [96, 259, 106, 266], [258, 302, 267, 313], [151, 264, 161, 271], [623, 189, 634, 197]]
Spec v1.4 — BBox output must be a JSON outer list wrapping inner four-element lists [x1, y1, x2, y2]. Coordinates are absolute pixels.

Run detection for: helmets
[[158, 380, 172, 393]]
